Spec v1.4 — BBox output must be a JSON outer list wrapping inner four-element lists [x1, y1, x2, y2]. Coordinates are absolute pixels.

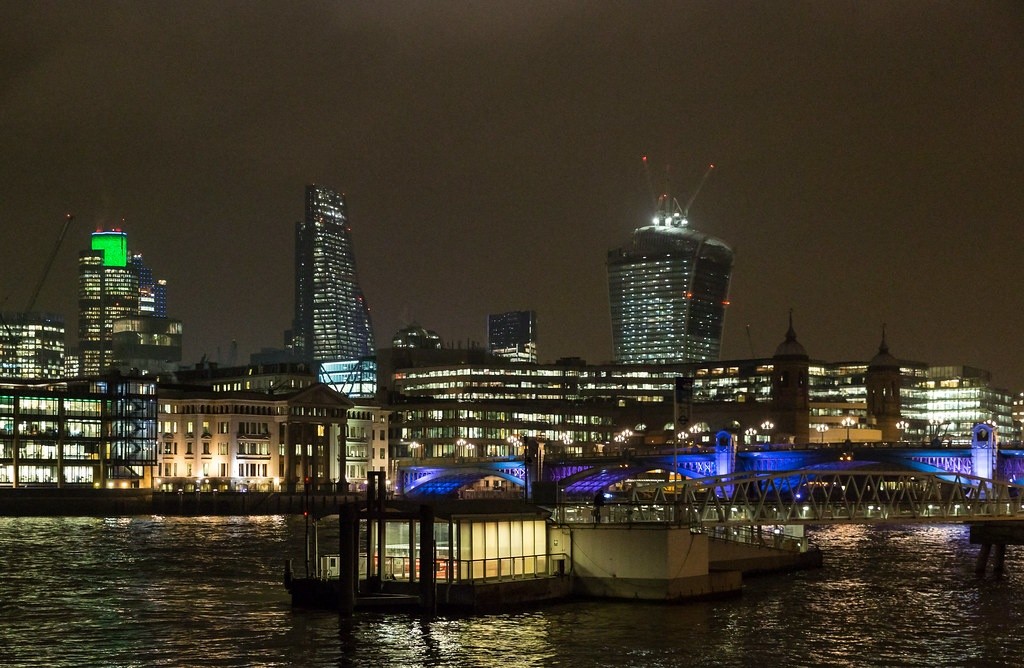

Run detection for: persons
[[593, 490, 606, 506]]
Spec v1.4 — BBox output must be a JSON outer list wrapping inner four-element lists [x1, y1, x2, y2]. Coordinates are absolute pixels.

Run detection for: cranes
[[0, 212, 75, 320]]
